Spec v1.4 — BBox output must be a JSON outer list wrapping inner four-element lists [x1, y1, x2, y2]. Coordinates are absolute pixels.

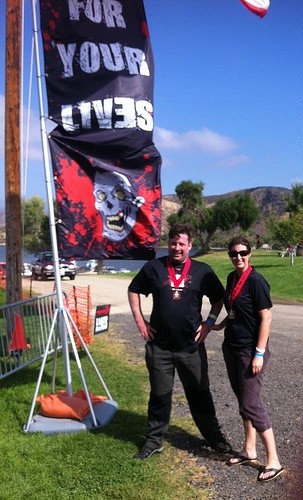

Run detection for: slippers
[[258, 467, 285, 482], [226, 454, 257, 466]]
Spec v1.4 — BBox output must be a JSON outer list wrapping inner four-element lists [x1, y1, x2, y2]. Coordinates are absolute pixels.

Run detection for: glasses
[[228, 250, 249, 257]]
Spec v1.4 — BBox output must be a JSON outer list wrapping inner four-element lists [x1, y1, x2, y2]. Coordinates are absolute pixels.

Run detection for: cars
[[0, 262, 31, 278], [94, 264, 131, 274]]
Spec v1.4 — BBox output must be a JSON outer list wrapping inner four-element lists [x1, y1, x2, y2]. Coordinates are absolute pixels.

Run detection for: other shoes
[[211, 439, 232, 453], [133, 445, 164, 459]]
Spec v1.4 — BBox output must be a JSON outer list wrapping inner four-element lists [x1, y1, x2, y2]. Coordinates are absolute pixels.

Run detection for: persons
[[128, 225, 231, 459], [288, 243, 295, 266], [209, 237, 284, 481]]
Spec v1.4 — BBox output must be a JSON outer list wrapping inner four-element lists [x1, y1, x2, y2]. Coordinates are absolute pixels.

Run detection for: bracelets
[[206, 314, 216, 327], [255, 347, 265, 356]]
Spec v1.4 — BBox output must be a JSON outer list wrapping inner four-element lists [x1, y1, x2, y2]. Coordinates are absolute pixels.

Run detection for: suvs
[[30, 250, 77, 280]]
